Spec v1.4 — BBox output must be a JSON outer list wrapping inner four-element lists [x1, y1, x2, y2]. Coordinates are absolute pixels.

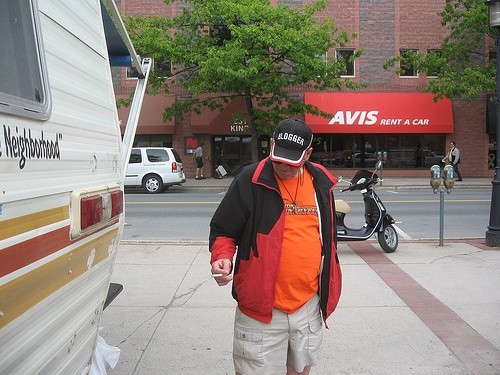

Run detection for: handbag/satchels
[[197, 157, 204, 167], [452, 155, 461, 164]]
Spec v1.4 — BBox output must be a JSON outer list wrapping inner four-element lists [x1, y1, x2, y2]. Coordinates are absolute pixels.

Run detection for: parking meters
[[376, 151, 388, 185]]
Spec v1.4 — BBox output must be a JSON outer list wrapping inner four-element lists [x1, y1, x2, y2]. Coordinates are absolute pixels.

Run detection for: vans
[[1, 1, 159, 375], [124, 147, 187, 193]]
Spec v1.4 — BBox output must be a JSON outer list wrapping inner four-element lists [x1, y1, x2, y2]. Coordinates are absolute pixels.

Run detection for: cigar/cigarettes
[[210, 273, 225, 277]]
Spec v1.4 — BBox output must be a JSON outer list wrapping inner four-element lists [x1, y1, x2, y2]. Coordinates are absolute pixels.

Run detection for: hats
[[269, 118, 313, 167]]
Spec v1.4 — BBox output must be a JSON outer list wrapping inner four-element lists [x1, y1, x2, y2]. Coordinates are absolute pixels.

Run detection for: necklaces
[[275, 165, 301, 211]]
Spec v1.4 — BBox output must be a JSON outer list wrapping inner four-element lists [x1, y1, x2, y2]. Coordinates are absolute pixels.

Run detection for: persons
[[191, 141, 206, 179], [351, 140, 425, 169], [212, 149, 223, 179], [210, 118, 344, 374], [441, 141, 465, 182]]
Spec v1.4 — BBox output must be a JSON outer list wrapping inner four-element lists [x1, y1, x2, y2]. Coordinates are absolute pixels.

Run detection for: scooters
[[334, 160, 399, 254]]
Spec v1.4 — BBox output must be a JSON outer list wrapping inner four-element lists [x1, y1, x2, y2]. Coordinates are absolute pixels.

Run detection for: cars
[[309, 148, 438, 169]]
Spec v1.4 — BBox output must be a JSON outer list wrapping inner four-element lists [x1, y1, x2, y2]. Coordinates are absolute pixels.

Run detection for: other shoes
[[455, 180, 462, 181], [196, 177, 202, 181], [200, 176, 206, 180]]
